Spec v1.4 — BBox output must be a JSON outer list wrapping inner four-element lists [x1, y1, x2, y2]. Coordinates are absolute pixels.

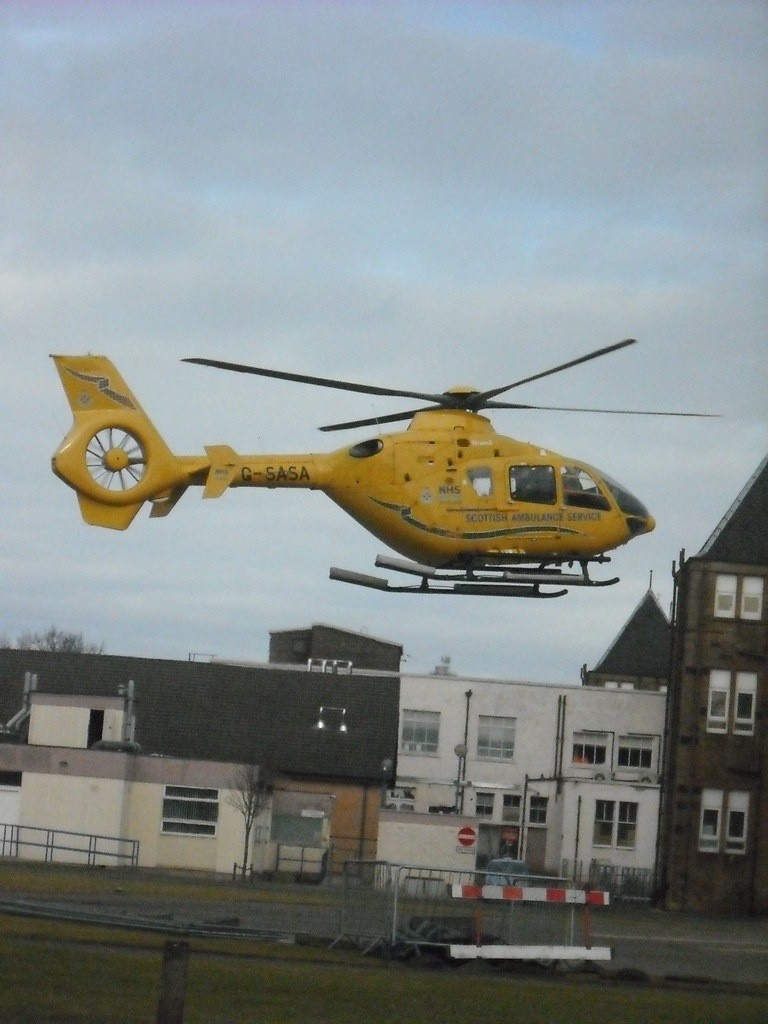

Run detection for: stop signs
[[458, 827, 475, 846]]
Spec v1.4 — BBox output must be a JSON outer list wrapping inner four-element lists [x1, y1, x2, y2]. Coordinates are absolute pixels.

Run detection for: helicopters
[[47, 337, 723, 598]]
